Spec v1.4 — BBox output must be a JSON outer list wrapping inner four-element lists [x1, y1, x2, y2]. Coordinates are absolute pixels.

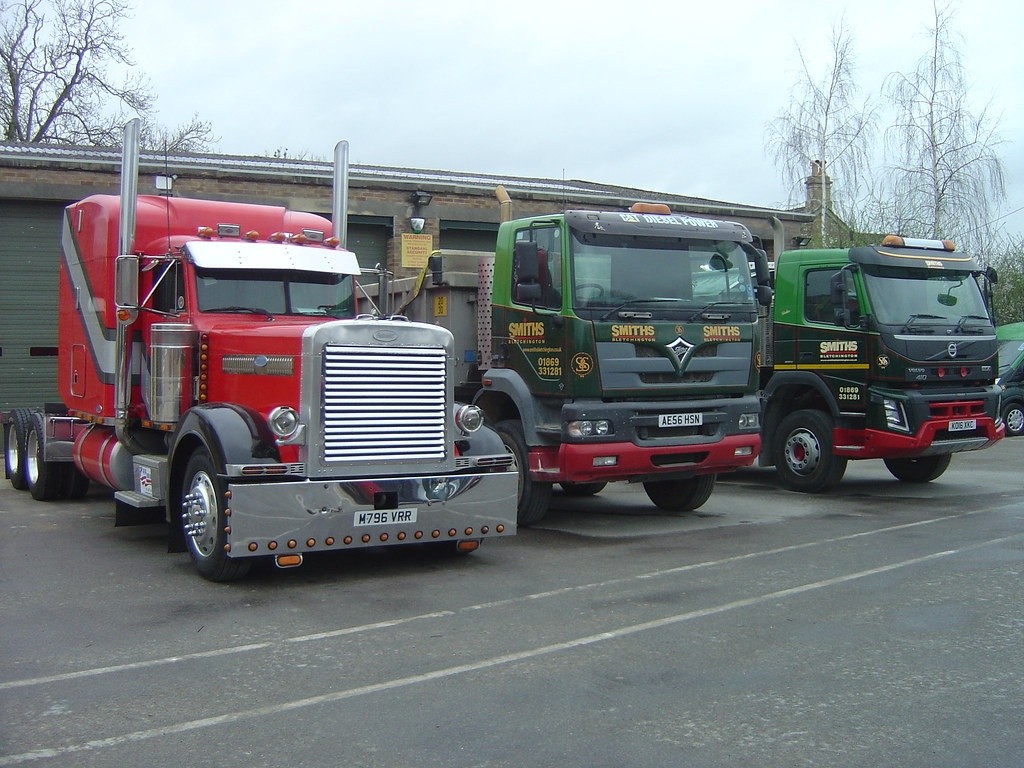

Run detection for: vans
[[995, 321, 1024, 436]]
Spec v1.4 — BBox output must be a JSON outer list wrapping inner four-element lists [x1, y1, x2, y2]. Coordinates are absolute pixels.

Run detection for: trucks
[[1, 118, 520, 585], [353, 185, 779, 526], [689, 214, 1006, 494]]
